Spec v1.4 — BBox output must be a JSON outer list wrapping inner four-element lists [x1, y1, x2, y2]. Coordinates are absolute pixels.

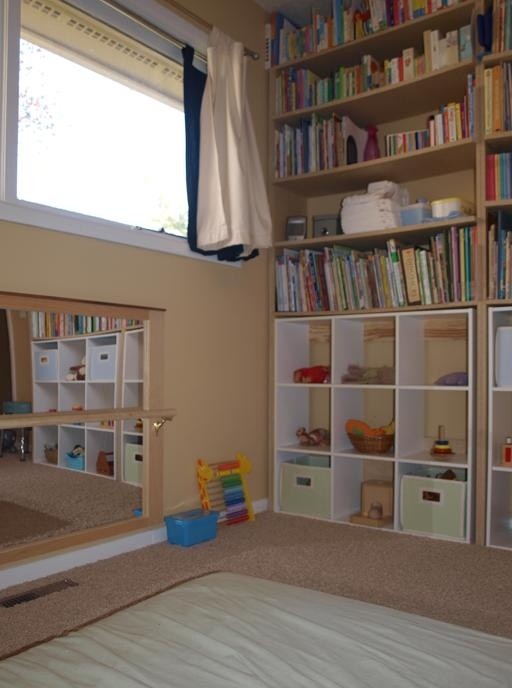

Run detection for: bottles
[[499, 436, 512, 466], [364, 119, 381, 160]]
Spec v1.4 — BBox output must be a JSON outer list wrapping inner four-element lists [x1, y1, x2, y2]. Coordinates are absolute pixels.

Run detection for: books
[[484, 62, 511, 133], [485, 152, 511, 200], [30, 311, 142, 339], [276, 25, 474, 116], [276, 1, 463, 65], [488, 224, 511, 299], [276, 226, 476, 312], [274, 75, 475, 180], [492, 1, 512, 53]]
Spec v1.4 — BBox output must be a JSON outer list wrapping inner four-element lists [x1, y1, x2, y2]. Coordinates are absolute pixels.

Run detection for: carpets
[[0, 570, 512, 688]]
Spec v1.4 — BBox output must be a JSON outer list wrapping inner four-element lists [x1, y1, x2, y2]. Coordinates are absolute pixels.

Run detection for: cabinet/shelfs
[[263, 1, 512, 552], [27, 310, 146, 488]]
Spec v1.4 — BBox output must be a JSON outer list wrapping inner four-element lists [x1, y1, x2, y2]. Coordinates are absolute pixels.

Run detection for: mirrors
[[0, 293, 167, 567]]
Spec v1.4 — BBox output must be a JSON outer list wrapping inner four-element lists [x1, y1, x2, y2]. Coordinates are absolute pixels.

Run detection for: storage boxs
[[162, 509, 220, 548]]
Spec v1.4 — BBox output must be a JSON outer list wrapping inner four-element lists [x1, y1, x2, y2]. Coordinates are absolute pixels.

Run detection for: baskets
[[347, 432, 393, 456]]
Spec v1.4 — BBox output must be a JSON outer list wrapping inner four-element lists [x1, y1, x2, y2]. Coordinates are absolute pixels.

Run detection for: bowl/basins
[[345, 433, 394, 453]]
